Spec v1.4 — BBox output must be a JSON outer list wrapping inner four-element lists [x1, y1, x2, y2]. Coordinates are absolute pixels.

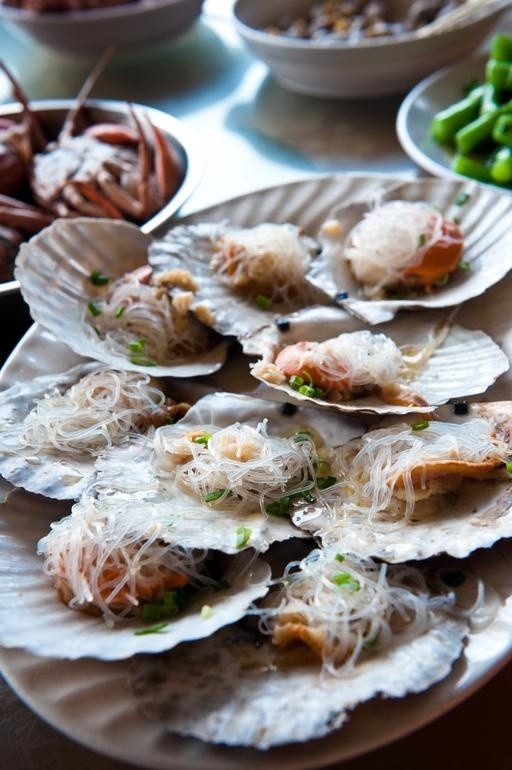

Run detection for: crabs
[[0, 47, 179, 234]]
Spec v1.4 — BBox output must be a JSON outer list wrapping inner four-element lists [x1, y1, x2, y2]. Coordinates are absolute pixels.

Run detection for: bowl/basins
[[229, 1, 508, 99], [0, 95, 195, 300], [0, 1, 208, 51]]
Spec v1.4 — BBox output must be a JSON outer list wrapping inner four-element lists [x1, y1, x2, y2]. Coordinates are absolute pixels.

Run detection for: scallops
[[1, 180, 512, 754]]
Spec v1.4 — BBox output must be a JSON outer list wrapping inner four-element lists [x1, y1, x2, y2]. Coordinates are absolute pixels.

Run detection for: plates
[[0, 170, 510, 768], [399, 56, 510, 191]]
